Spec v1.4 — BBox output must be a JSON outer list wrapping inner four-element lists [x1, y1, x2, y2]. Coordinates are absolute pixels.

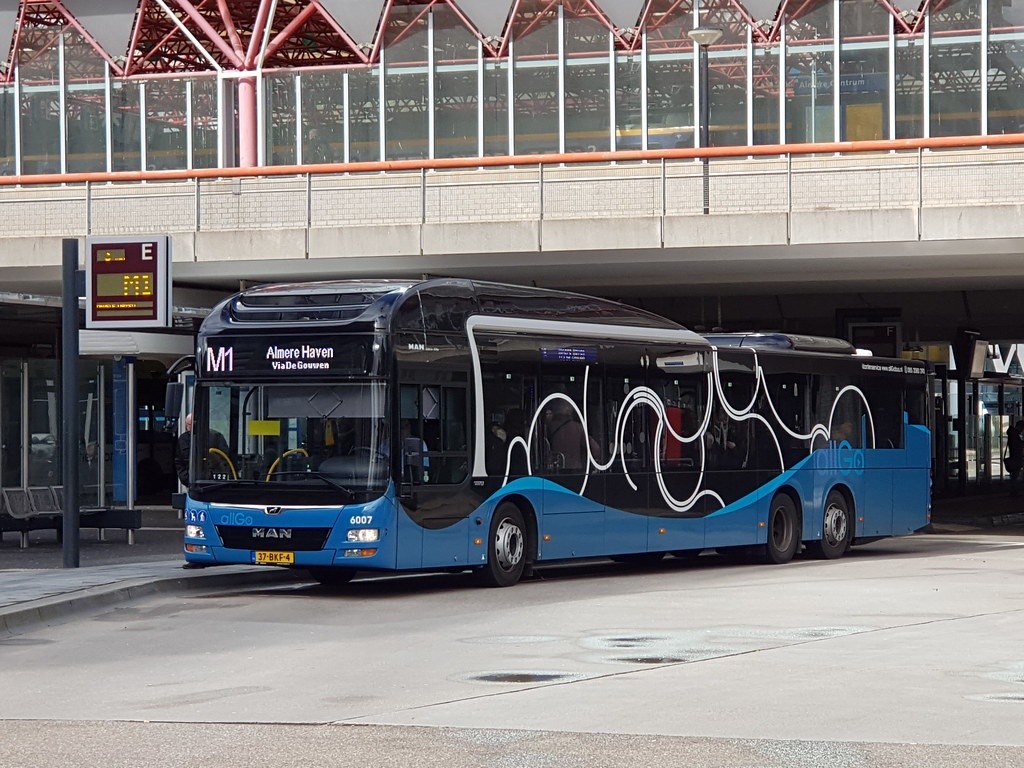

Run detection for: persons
[[545, 396, 602, 475], [706, 403, 747, 469], [1006, 420, 1024, 497], [375, 418, 430, 485], [173, 413, 231, 569]]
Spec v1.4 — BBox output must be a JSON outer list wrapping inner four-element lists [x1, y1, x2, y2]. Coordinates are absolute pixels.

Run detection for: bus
[[168, 281, 934, 587]]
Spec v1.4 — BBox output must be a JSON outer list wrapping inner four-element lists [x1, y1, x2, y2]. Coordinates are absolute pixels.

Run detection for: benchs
[[0, 485, 107, 520]]
